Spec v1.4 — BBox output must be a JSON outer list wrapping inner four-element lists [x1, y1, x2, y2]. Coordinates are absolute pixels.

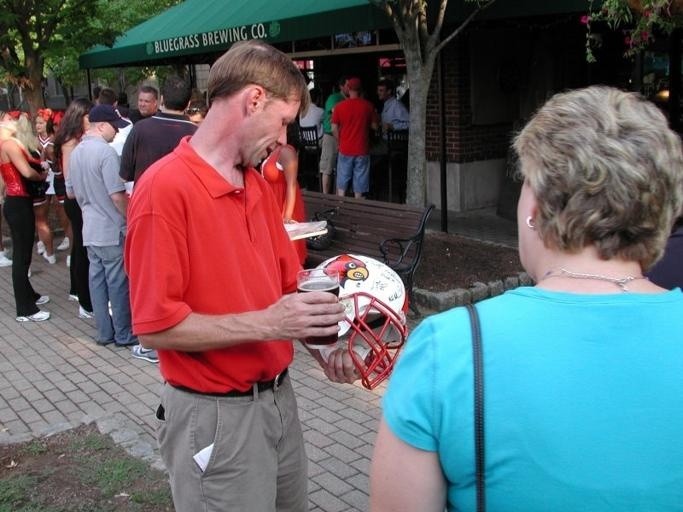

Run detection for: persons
[[302, 73, 409, 198], [125, 39, 360, 510], [367, 84, 682, 512], [2, 74, 208, 362]]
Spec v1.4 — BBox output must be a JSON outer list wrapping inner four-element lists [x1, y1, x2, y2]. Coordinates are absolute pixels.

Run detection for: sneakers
[[0, 237, 159, 363]]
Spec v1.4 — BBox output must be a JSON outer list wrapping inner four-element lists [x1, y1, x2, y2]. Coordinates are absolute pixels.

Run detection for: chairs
[[384, 127, 408, 203], [298, 125, 323, 194]]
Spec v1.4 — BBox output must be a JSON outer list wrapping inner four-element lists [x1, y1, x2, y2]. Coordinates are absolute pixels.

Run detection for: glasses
[[185, 108, 199, 115]]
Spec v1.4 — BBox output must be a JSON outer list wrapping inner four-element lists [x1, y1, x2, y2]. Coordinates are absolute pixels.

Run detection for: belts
[[167, 368, 288, 398]]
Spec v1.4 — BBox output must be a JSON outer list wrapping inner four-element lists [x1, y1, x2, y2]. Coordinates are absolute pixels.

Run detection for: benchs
[[297, 187, 436, 320]]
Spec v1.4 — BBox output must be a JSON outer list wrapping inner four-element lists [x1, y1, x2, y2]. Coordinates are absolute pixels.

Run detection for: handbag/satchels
[[23, 162, 49, 195], [307, 209, 337, 249]]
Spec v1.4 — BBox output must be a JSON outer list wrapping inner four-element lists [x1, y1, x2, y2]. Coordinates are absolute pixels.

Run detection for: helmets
[[301, 252, 408, 389]]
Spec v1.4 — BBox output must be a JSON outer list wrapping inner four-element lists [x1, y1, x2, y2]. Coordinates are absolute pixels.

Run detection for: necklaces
[[537, 263, 651, 292]]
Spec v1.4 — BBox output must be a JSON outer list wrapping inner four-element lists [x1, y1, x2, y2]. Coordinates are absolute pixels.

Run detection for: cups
[[297, 267, 338, 348]]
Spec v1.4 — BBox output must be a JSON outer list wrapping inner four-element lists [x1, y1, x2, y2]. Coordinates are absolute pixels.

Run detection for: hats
[[88, 106, 130, 129], [347, 79, 360, 90]]
